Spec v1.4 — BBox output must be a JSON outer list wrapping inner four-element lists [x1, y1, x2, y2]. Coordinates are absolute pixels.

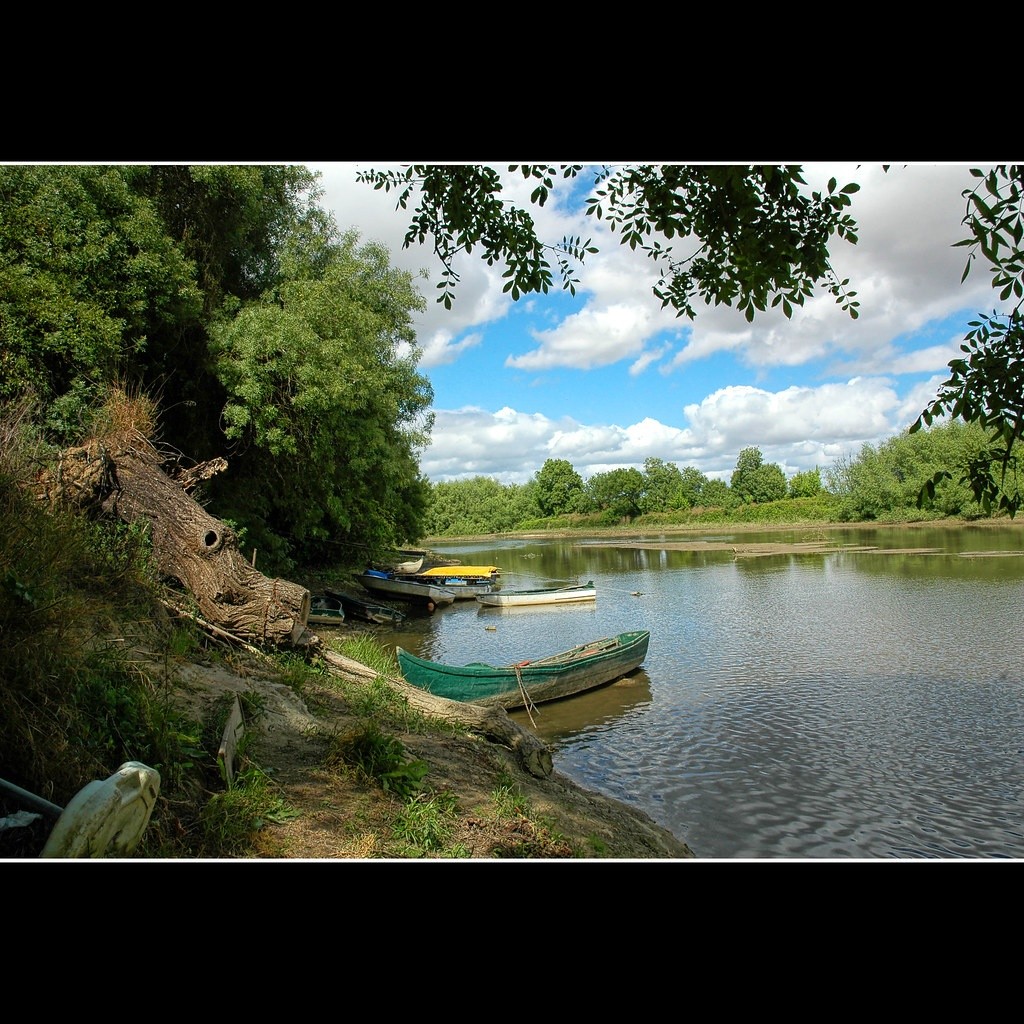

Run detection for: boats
[[475, 582, 596, 604], [342, 582, 406, 627], [393, 631, 650, 712], [351, 571, 455, 604], [391, 566, 497, 599], [310, 594, 347, 627]]
